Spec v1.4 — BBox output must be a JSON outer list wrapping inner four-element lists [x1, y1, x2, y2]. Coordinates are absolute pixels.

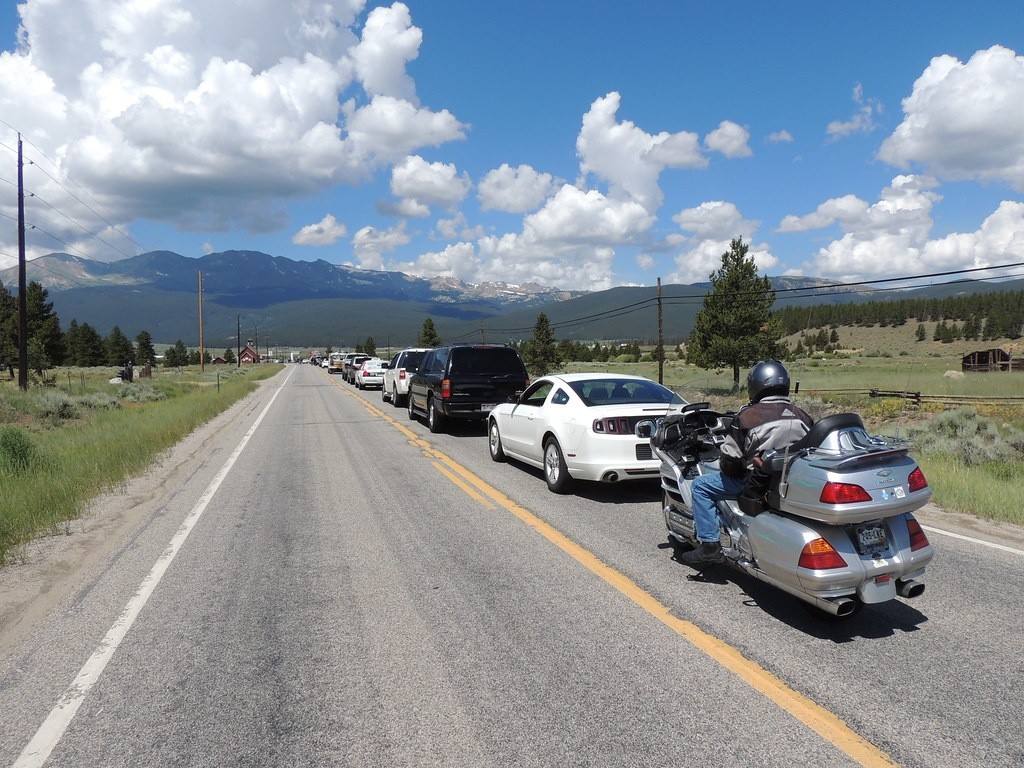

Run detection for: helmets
[[747, 358, 791, 406]]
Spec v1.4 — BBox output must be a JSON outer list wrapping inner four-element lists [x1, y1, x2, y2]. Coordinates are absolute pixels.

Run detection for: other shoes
[[683, 540, 725, 564]]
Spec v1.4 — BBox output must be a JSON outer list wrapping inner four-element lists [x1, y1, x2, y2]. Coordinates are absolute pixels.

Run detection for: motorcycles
[[636, 377, 932, 618]]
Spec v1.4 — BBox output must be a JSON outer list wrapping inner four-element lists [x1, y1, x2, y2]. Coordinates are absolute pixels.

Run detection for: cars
[[355, 358, 391, 390], [340, 353, 373, 385], [291, 355, 329, 369], [488, 372, 713, 494]]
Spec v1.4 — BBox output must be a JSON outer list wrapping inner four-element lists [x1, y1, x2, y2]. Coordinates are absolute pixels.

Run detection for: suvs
[[381, 348, 434, 407], [407, 342, 531, 434]]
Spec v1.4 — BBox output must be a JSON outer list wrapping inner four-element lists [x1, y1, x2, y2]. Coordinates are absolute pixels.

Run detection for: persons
[[681, 361, 812, 565]]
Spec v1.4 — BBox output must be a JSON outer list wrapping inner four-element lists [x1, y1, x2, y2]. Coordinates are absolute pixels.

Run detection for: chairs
[[611, 387, 631, 401], [589, 387, 609, 400], [633, 387, 651, 398]]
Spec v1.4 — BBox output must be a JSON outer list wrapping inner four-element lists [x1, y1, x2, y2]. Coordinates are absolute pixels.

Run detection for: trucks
[[328, 353, 347, 374]]
